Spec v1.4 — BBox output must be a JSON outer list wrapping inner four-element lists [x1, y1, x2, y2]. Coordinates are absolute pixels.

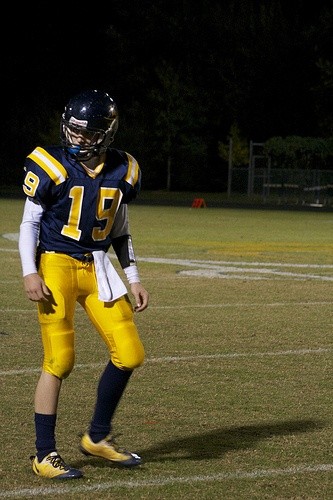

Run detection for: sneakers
[[80, 425, 142, 466], [30, 450, 84, 482]]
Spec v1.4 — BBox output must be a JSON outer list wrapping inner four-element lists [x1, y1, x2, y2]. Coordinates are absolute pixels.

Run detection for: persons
[[18, 89, 150, 480]]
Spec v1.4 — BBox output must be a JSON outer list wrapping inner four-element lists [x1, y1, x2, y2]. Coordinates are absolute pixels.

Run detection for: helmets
[[61, 89, 118, 160]]
